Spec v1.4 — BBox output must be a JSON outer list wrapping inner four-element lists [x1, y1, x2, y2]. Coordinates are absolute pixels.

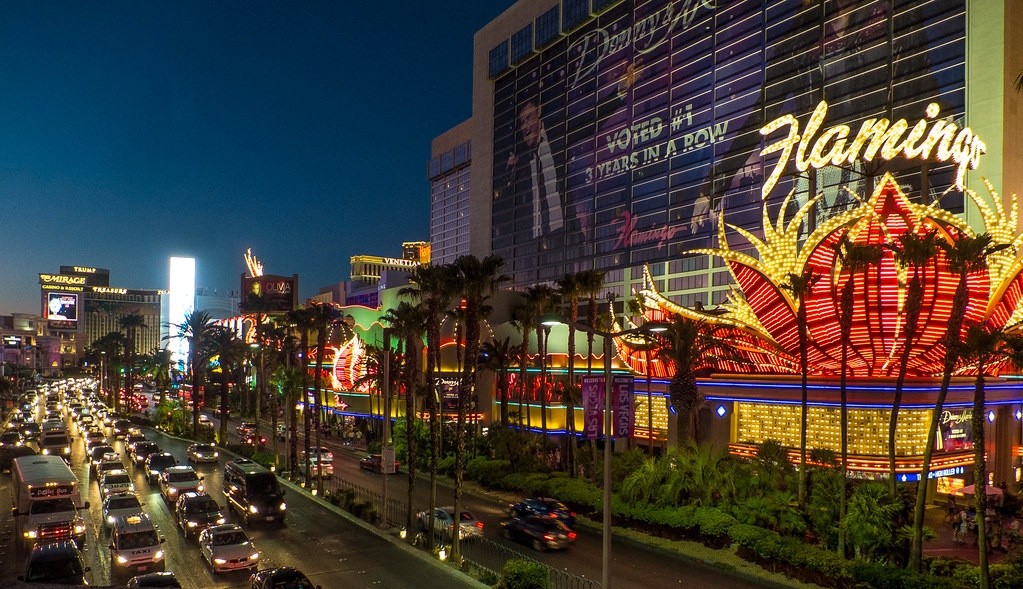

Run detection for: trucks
[[221, 458, 287, 531], [12, 455, 92, 552]]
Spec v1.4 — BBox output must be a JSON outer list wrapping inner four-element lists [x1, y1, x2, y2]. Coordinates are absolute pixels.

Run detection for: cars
[[298, 446, 334, 480], [199, 524, 260, 576], [360, 454, 400, 475], [97, 452, 129, 482], [176, 491, 225, 540], [0, 377, 322, 589], [102, 492, 149, 530], [416, 506, 485, 544], [89, 446, 120, 473], [240, 433, 266, 446], [185, 442, 219, 464], [99, 469, 135, 500], [499, 498, 577, 553], [124, 429, 147, 454], [130, 440, 163, 468], [112, 419, 134, 439]]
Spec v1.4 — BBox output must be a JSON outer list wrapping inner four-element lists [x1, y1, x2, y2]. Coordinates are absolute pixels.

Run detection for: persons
[[547, 448, 560, 468], [987, 494, 1004, 516], [121, 533, 154, 547], [985, 516, 993, 554], [943, 494, 979, 545], [250, 282, 263, 297], [503, 58, 644, 283], [999, 481, 1008, 494], [213, 533, 236, 545], [692, 0, 942, 242], [356, 429, 362, 446], [365, 429, 371, 446], [337, 421, 355, 442], [1006, 515, 1020, 550]]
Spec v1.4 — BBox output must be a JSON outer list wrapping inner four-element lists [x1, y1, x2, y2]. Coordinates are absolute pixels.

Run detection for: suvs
[[213, 405, 230, 418], [109, 513, 167, 580], [157, 465, 205, 507], [236, 422, 259, 435], [144, 452, 179, 485], [17, 539, 92, 585], [276, 425, 299, 443]]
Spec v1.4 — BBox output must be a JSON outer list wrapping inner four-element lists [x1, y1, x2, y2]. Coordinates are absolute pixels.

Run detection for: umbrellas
[[958, 484, 1002, 494]]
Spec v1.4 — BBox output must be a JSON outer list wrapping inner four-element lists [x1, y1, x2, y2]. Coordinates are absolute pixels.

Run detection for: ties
[[533, 149, 551, 237]]
